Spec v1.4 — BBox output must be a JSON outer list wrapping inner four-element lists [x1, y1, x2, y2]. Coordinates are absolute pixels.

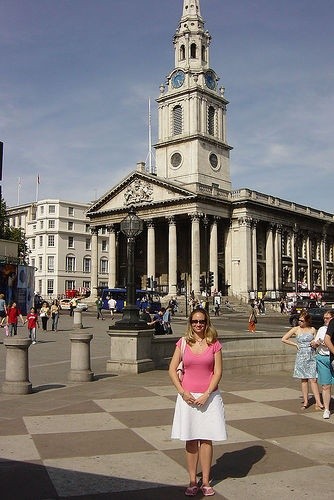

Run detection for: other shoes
[[32, 341, 36, 344], [315, 405, 324, 410], [301, 402, 310, 409]]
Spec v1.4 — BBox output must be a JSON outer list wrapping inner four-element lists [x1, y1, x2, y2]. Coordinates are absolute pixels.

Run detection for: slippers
[[199, 484, 215, 496], [185, 486, 199, 496]]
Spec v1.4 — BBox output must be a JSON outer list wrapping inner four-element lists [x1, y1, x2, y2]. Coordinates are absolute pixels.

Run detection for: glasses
[[190, 320, 206, 324], [323, 316, 334, 320]]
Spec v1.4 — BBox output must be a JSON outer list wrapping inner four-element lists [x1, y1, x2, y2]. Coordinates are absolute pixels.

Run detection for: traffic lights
[[207, 287, 211, 295], [207, 271, 214, 286], [200, 274, 205, 290], [146, 278, 150, 288]]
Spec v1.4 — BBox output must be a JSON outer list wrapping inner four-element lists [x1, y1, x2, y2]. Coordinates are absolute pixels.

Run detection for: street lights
[[108, 205, 151, 331]]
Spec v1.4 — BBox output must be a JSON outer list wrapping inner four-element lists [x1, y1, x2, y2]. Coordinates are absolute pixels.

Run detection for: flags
[[38, 177, 40, 184]]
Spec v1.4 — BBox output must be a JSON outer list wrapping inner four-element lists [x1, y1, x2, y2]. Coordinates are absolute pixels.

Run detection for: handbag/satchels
[[4, 324, 10, 337], [176, 337, 188, 382], [254, 319, 257, 323]]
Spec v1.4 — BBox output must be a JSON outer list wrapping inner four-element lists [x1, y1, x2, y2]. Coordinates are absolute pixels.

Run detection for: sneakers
[[323, 409, 330, 419]]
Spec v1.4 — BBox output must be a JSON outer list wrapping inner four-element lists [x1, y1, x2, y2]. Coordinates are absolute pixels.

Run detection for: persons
[[248, 304, 257, 333], [24, 307, 40, 344], [188, 289, 223, 317], [143, 306, 171, 335], [169, 308, 228, 496], [280, 292, 322, 313], [40, 299, 59, 332], [166, 296, 178, 317], [0, 294, 7, 328], [6, 302, 25, 336], [108, 296, 117, 320], [253, 298, 265, 315], [95, 296, 104, 320], [281, 309, 334, 419], [68, 296, 81, 317]]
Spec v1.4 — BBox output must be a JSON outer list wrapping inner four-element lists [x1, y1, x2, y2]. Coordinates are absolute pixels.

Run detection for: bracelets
[[182, 392, 185, 397]]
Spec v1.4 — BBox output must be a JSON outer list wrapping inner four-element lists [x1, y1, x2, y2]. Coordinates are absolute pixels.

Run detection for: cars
[[288, 308, 334, 331], [58, 299, 88, 311], [290, 301, 319, 314]]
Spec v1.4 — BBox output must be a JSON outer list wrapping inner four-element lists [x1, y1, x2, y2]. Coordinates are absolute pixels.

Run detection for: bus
[[101, 287, 162, 314]]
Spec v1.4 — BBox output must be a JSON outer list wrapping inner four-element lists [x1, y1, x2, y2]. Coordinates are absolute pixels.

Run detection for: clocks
[[205, 72, 216, 89], [172, 72, 185, 88]]
